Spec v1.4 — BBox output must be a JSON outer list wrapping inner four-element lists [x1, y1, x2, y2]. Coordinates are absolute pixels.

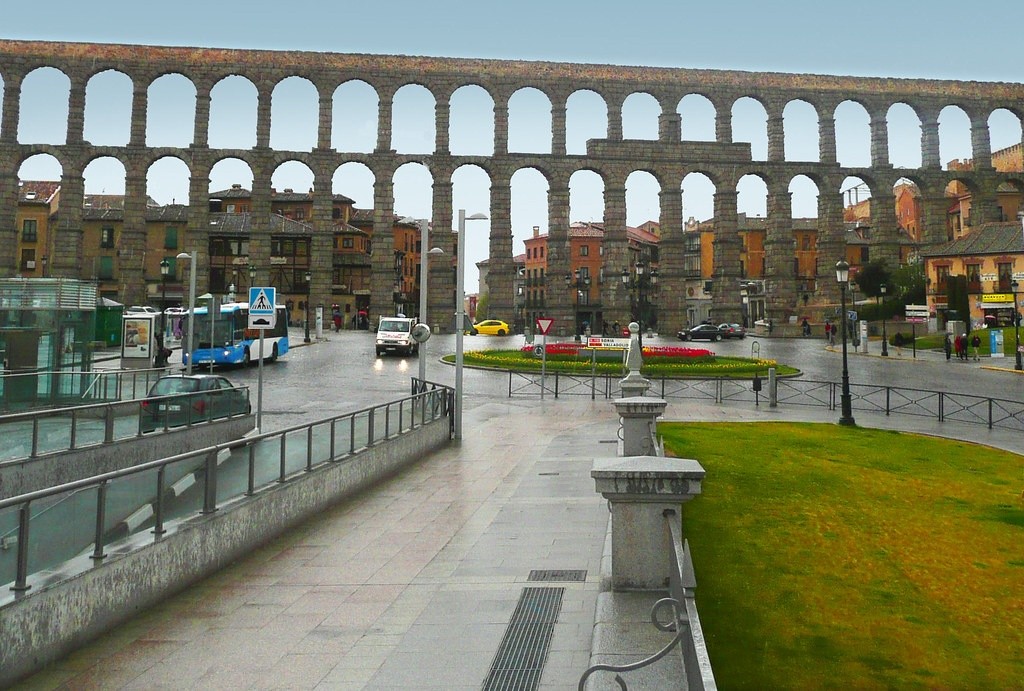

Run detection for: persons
[[401, 322, 408, 330], [960, 333, 969, 360], [799, 317, 808, 335], [612, 320, 620, 333], [768, 319, 773, 335], [176, 379, 193, 392], [628, 314, 636, 322], [824, 321, 830, 339], [830, 324, 837, 345], [332, 310, 342, 332], [351, 314, 369, 329], [943, 335, 951, 359], [894, 331, 903, 355], [971, 333, 981, 361], [821, 318, 835, 323], [954, 336, 961, 357], [601, 319, 609, 337], [391, 323, 400, 331], [153, 329, 169, 363]]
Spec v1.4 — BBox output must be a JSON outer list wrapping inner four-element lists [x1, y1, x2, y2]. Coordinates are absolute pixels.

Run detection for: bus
[[178, 303, 290, 371]]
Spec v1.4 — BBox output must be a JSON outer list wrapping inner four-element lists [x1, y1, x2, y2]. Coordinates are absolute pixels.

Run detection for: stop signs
[[622, 328, 630, 337]]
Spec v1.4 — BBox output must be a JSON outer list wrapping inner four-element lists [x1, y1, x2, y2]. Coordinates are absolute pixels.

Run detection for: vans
[[375, 315, 419, 357]]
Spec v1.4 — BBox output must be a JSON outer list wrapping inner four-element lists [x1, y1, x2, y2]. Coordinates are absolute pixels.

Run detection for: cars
[[718, 322, 746, 340], [676, 323, 728, 342], [465, 319, 510, 337], [139, 374, 252, 434]]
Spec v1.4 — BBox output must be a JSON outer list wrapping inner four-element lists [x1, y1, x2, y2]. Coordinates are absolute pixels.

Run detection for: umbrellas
[[941, 331, 954, 335], [985, 315, 996, 318]]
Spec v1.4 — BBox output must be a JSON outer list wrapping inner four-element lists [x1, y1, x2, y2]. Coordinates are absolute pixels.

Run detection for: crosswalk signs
[[249, 287, 276, 316]]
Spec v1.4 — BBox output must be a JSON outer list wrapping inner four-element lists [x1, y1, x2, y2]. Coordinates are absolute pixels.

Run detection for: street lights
[[454, 210, 489, 440], [834, 258, 856, 426], [153, 257, 170, 371], [879, 283, 888, 356], [1009, 279, 1023, 370], [232, 267, 239, 296], [564, 268, 592, 342], [418, 219, 444, 393], [248, 266, 258, 288], [176, 250, 198, 376], [304, 271, 314, 342], [621, 259, 659, 351], [197, 293, 215, 375]]
